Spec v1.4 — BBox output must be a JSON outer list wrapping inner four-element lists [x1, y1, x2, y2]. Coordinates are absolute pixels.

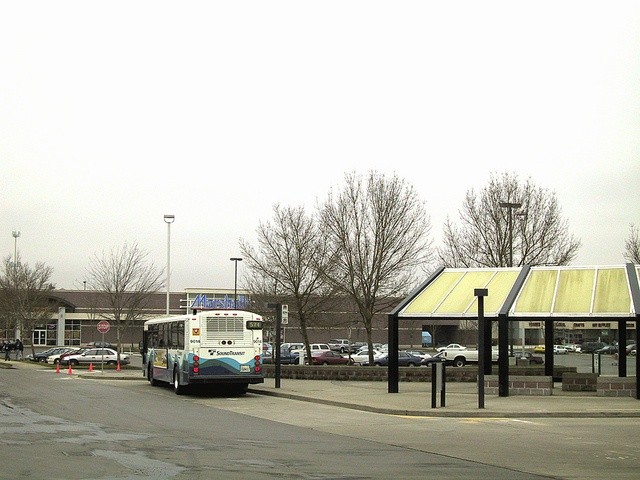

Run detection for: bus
[[139, 309, 264, 394]]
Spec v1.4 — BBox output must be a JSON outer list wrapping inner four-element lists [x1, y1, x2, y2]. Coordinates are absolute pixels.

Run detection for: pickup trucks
[[442, 346, 499, 368]]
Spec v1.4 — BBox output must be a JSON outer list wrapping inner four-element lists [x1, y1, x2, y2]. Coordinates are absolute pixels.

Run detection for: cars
[[61, 347, 130, 367], [507, 350, 515, 357], [626, 343, 636, 355], [553, 344, 568, 355], [420, 351, 453, 367], [631, 345, 637, 355], [343, 349, 385, 363], [311, 349, 355, 366], [59, 346, 99, 361], [594, 345, 618, 355], [28, 347, 58, 361], [534, 345, 546, 354], [516, 351, 544, 365], [45, 349, 81, 365], [35, 348, 60, 364], [360, 351, 421, 367], [274, 349, 299, 365], [435, 343, 467, 353], [564, 343, 582, 352], [406, 350, 431, 362]]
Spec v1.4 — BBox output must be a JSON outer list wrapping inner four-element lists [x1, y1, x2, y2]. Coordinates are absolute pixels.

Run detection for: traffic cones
[[88, 361, 93, 371], [67, 365, 72, 375], [116, 360, 121, 371], [56, 364, 60, 373]]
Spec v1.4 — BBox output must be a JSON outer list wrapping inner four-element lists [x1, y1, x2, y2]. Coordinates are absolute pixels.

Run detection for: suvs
[[329, 339, 352, 354], [290, 343, 332, 358]]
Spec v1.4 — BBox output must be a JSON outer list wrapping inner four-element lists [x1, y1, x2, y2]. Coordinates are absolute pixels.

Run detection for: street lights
[[230, 258, 242, 308], [165, 215, 174, 315], [12, 231, 21, 290]]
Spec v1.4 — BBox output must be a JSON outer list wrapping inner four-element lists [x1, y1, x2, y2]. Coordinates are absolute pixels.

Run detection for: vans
[[581, 341, 609, 353], [280, 342, 304, 349]]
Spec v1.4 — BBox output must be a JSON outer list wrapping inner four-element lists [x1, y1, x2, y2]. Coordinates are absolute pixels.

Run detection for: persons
[[2, 341, 12, 361], [612, 353, 619, 366], [14, 339, 23, 361]]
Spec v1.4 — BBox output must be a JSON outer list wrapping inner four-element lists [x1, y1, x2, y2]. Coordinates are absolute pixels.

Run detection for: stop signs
[[97, 320, 111, 333]]
